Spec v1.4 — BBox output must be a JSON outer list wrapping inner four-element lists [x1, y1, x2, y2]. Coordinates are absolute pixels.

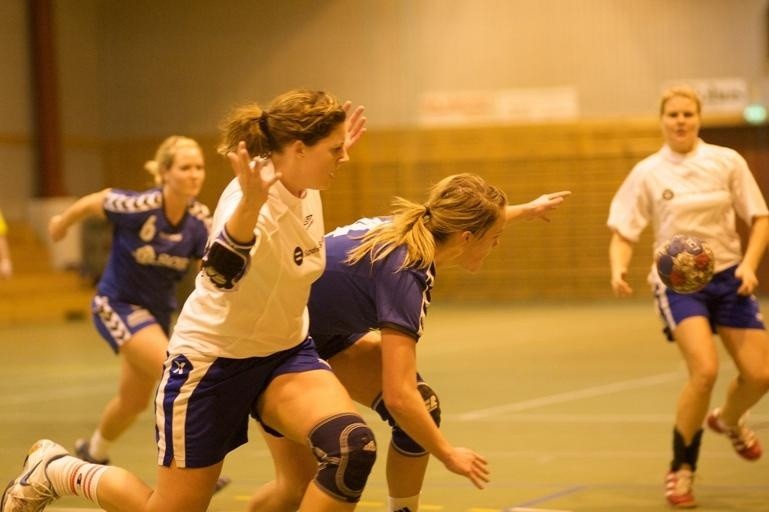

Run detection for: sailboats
[[655, 236, 715, 295]]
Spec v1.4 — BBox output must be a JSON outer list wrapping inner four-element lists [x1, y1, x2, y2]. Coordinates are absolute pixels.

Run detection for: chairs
[[75, 436, 111, 466], [707, 407, 762, 461], [0, 438, 71, 511], [666, 463, 696, 507]]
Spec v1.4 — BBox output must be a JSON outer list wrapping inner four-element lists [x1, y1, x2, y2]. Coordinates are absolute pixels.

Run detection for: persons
[[248, 173, 574, 512], [0, 86, 377, 512], [45, 131, 234, 497], [603, 92, 769, 508]]
